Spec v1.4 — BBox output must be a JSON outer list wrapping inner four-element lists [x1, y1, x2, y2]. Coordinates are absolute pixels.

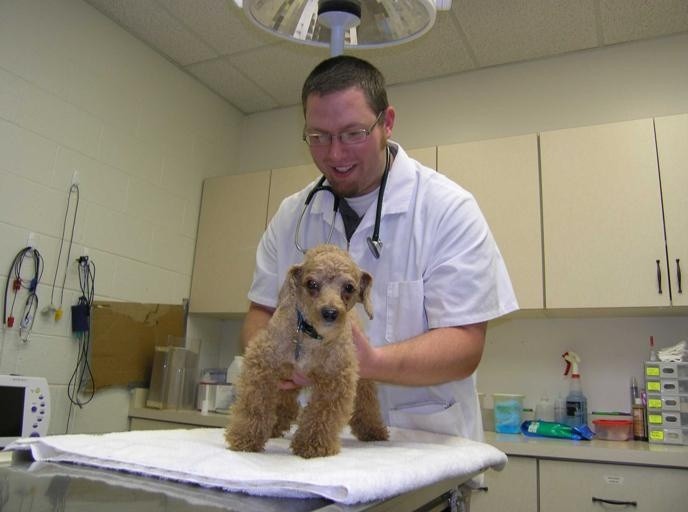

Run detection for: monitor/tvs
[[0, 376, 51, 448]]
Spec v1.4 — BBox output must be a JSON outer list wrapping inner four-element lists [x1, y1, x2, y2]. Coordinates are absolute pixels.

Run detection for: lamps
[[243, 1, 437, 59]]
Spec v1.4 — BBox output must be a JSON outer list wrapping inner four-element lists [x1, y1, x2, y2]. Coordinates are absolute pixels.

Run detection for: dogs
[[224, 244, 389, 460]]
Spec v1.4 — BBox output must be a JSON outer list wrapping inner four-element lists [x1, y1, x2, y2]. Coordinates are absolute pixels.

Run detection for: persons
[[241, 55, 519, 489]]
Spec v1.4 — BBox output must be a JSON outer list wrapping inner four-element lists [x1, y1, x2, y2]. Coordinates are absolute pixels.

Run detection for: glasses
[[302, 111, 383, 147]]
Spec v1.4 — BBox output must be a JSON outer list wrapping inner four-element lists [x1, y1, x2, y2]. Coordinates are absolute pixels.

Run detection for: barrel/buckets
[[492, 392, 527, 436]]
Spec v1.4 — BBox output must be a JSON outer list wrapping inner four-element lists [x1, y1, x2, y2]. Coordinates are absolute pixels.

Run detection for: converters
[[70, 304, 89, 332]]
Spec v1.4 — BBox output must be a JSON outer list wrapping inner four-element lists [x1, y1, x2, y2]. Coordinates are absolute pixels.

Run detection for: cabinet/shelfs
[[538, 442, 688, 511], [540, 112, 688, 318], [127, 407, 228, 432], [403, 132, 548, 321], [469, 436, 538, 510], [187, 164, 324, 321]]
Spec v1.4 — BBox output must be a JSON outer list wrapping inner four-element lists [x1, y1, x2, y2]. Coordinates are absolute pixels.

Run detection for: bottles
[[226, 355, 244, 383], [536, 389, 565, 424], [632, 397, 647, 441]]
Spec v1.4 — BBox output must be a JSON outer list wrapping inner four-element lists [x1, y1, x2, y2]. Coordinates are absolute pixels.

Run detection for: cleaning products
[[533, 388, 556, 423], [561, 349, 587, 425]]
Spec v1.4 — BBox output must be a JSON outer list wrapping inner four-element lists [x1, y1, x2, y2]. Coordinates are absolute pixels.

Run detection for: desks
[[0, 461, 475, 512]]
